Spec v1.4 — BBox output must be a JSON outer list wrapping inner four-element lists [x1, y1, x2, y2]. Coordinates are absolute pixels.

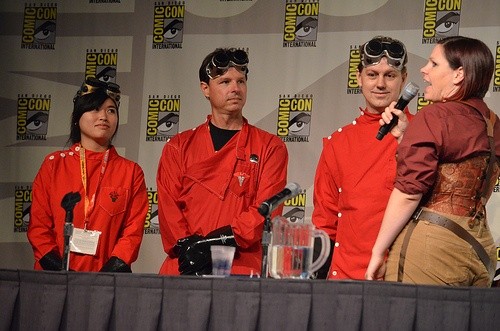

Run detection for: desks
[[0, 267, 500, 331]]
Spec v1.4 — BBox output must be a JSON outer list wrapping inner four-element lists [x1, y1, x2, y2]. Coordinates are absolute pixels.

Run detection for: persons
[[364, 36, 500, 289], [312, 35, 415, 280], [27, 78, 149, 273], [156, 47, 288, 277]]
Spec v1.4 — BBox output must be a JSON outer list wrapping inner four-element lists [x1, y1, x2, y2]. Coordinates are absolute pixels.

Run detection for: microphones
[[257, 182, 301, 216], [375, 82, 420, 141]]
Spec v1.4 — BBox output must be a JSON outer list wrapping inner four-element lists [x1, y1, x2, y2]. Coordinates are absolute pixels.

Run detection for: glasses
[[206, 50, 249, 79], [360, 37, 406, 71], [80, 78, 120, 108]]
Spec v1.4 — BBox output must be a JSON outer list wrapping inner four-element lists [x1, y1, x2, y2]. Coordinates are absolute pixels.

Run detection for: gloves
[[303, 237, 335, 279], [174, 225, 238, 275]]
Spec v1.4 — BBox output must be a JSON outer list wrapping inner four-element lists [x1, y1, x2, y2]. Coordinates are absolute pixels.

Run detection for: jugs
[[267, 216, 331, 279]]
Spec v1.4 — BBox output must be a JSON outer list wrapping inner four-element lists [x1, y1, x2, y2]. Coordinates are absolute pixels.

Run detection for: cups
[[211, 246, 236, 276]]
